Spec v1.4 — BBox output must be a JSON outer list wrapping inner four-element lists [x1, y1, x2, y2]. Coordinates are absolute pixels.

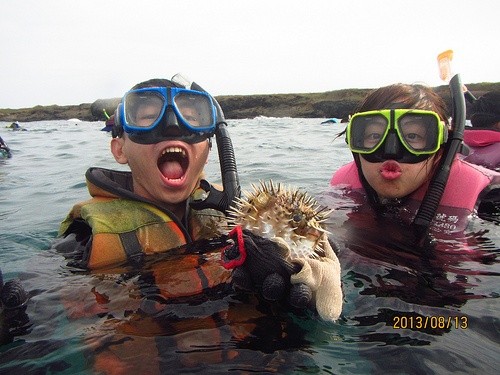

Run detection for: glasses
[[119, 87, 216, 133], [346, 109, 443, 155]]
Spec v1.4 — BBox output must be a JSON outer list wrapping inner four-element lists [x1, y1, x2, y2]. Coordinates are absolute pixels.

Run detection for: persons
[[0, 78, 337, 375], [323, 84, 500, 374]]
